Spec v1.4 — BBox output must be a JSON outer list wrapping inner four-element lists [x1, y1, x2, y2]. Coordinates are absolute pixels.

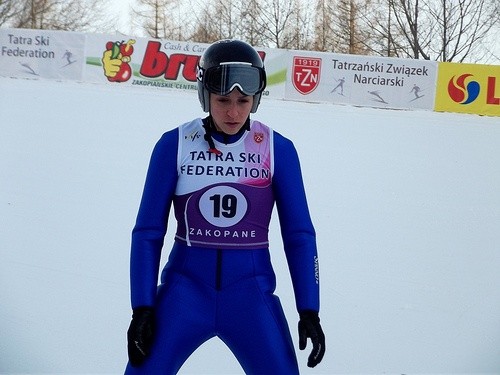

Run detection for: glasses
[[196, 63, 267, 97]]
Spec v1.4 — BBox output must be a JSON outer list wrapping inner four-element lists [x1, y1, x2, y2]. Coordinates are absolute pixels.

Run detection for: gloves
[[298, 311, 326, 368], [127, 308, 157, 367]]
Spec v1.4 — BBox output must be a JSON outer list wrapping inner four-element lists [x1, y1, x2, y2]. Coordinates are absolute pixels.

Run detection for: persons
[[123, 39, 325, 375]]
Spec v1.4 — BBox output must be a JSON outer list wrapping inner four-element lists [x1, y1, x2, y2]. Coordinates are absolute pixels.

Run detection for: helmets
[[196, 39, 264, 113]]
